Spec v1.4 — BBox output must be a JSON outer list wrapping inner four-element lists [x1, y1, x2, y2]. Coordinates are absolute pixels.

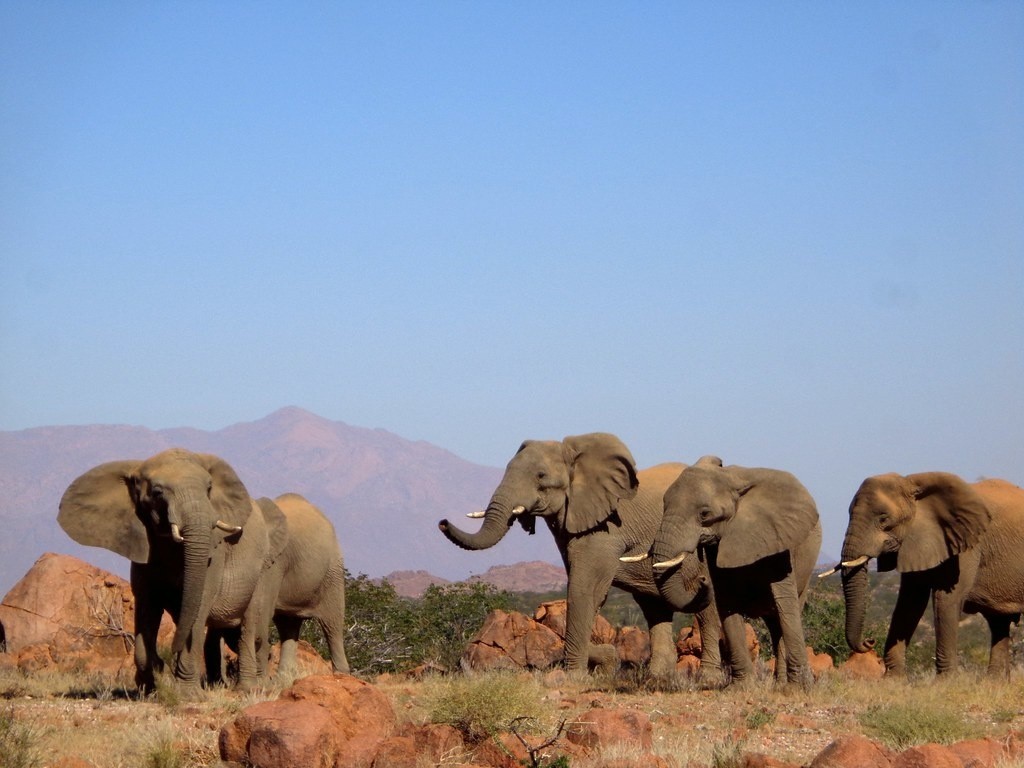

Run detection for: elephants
[[817, 470, 1023, 689], [56, 447, 351, 699], [437, 431, 823, 693]]
